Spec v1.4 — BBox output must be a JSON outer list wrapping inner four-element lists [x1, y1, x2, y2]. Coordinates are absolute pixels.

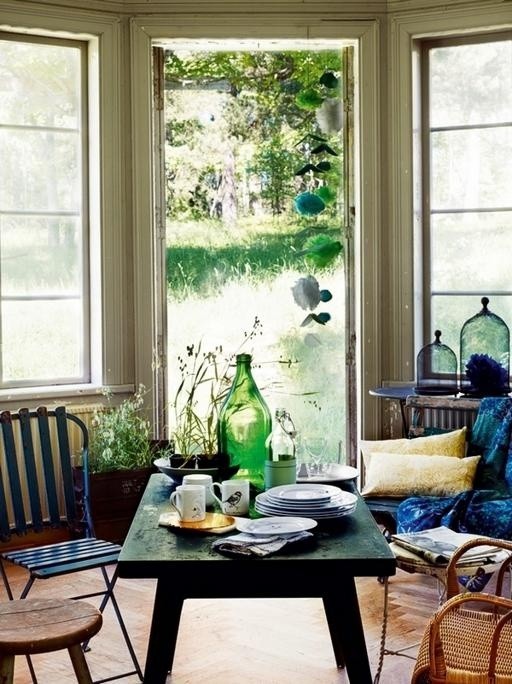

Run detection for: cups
[[170, 485, 206, 522], [209, 478, 251, 516], [182, 474, 215, 508]]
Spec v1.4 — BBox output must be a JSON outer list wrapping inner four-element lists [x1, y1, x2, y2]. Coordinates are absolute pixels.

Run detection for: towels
[[212, 530, 314, 559]]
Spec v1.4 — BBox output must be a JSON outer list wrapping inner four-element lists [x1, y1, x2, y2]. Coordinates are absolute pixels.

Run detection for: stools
[[0, 598, 103, 684], [373, 529, 512, 684]]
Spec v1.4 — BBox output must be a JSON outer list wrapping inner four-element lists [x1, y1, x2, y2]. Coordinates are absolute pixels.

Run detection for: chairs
[[412, 538, 512, 684], [0, 406, 144, 684]]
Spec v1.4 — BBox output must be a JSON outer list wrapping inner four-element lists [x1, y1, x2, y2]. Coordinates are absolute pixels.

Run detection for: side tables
[[369, 386, 467, 439]]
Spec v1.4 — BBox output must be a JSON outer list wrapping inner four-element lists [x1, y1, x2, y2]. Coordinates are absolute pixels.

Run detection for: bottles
[[265, 408, 295, 487], [218, 352, 270, 499]]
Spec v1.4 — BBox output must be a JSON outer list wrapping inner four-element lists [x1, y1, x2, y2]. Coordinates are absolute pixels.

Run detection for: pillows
[[362, 426, 467, 465], [361, 452, 480, 499]]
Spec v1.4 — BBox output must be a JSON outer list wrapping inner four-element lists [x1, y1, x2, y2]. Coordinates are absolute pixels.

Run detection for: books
[[391, 526, 503, 566]]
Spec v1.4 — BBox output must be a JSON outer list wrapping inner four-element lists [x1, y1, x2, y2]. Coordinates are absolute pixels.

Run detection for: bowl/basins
[[153, 456, 240, 483]]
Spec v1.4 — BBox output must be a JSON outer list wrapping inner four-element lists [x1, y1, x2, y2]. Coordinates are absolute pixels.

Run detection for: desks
[[114, 470, 397, 684]]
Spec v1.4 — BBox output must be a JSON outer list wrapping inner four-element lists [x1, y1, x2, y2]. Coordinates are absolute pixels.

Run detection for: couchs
[[359, 394, 512, 594]]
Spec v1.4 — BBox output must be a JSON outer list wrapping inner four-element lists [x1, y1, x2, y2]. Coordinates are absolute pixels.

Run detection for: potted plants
[[145, 316, 322, 486], [50, 381, 171, 547]]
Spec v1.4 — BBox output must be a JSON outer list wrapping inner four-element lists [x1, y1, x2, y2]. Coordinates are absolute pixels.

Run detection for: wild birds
[[222, 491, 243, 509]]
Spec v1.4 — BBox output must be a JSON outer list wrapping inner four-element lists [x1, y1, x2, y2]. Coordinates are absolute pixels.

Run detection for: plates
[[253, 484, 358, 519], [295, 462, 360, 483], [237, 517, 319, 534]]
